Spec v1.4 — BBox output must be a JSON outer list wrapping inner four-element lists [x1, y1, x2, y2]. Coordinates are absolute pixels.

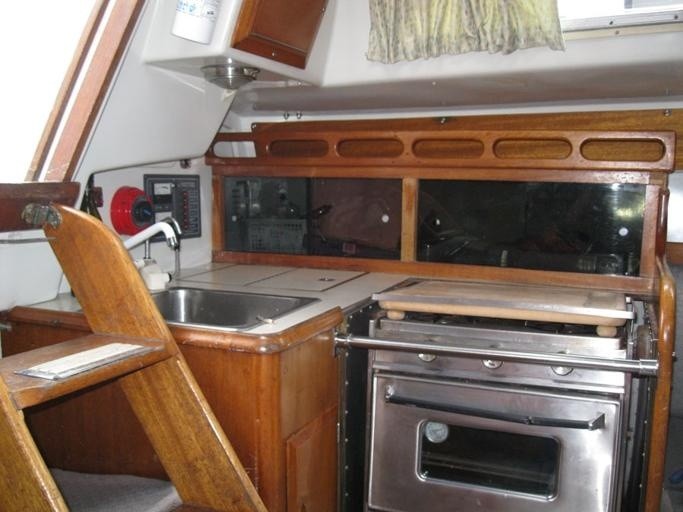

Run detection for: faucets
[[144, 215, 183, 258], [123, 222, 179, 249]]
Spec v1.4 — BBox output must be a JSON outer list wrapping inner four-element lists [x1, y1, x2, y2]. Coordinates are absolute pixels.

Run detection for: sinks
[[151, 289, 310, 330]]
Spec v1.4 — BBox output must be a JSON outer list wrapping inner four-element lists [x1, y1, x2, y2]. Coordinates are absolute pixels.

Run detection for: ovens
[[335, 309, 660, 512]]
